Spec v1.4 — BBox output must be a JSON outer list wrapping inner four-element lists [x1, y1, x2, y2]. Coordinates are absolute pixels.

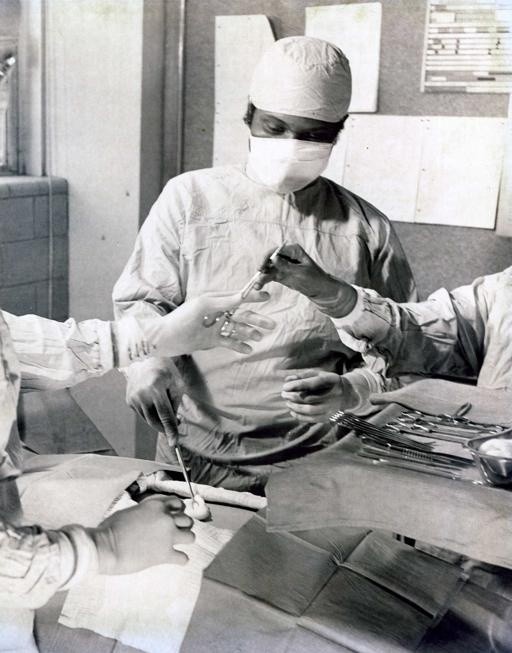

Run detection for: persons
[[254, 242, 512, 393], [111, 35, 420, 502], [0, 290, 274, 652]]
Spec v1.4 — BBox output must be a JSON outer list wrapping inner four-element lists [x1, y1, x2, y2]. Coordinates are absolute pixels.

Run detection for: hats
[[248, 35, 352, 123]]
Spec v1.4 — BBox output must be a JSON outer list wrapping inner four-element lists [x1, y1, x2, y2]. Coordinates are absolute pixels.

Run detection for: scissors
[[396, 409, 480, 432], [378, 419, 464, 445], [201, 237, 290, 339]]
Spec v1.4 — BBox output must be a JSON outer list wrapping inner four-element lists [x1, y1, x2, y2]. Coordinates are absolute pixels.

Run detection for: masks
[[242, 120, 333, 195]]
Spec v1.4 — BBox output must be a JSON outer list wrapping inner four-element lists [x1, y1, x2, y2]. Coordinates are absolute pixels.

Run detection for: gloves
[[84, 496, 196, 574], [135, 290, 276, 358], [253, 244, 357, 319]]
